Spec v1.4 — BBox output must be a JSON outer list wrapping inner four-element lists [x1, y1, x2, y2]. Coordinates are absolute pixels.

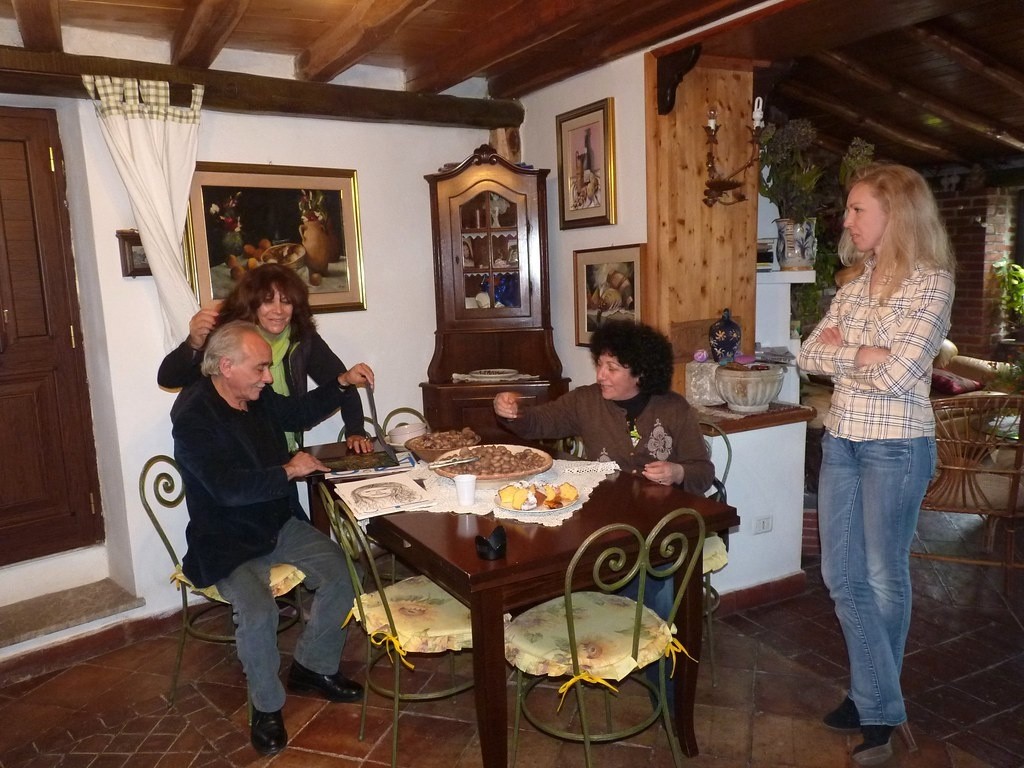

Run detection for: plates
[[469, 368, 518, 378], [494, 493, 581, 512]]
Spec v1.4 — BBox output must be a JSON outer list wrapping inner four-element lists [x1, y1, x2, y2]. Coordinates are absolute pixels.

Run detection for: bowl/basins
[[715, 363, 784, 411], [388, 422, 427, 444], [433, 444, 553, 490], [404, 433, 481, 464]]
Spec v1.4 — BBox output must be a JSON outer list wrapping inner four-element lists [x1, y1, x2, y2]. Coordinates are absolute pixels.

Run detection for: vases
[[773, 220, 817, 273]]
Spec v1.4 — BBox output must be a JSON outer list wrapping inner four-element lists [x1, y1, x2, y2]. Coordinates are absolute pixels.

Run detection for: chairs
[[297, 378, 386, 446], [314, 483, 510, 767], [500, 509, 704, 768], [703, 422, 730, 688], [911, 394, 1023, 611], [140, 455, 306, 712]]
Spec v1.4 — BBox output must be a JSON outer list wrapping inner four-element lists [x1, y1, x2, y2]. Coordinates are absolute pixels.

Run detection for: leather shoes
[[252, 706, 287, 754], [287, 659, 364, 702]]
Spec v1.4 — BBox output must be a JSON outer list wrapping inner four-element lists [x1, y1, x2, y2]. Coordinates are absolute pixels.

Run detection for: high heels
[[852, 722, 918, 766], [822, 695, 860, 730]]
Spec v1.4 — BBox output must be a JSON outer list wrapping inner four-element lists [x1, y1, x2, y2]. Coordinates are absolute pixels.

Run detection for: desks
[[294, 437, 741, 768]]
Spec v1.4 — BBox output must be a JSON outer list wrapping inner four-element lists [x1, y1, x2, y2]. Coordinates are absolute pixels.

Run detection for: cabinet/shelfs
[[421, 143, 574, 450]]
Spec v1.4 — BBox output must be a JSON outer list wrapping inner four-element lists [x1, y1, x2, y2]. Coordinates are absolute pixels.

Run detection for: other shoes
[[660, 712, 677, 737]]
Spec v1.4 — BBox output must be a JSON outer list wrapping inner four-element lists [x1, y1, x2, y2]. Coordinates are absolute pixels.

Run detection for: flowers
[[759, 118, 824, 221]]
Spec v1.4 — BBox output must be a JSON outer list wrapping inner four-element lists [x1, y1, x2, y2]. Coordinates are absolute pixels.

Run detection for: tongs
[[428, 456, 479, 470]]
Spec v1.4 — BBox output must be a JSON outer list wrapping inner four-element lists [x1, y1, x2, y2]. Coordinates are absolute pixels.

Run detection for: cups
[[452, 474, 477, 508]]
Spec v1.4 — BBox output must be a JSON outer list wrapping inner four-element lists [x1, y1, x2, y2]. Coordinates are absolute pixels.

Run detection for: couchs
[[804, 338, 1023, 465]]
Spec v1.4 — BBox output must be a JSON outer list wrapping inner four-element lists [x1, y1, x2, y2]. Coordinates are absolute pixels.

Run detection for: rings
[[659, 479, 662, 484], [363, 439, 371, 442]]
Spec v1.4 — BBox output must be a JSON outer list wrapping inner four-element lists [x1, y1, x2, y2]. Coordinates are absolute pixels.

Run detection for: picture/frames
[[555, 96, 617, 230], [574, 242, 647, 347], [188, 163, 366, 317]]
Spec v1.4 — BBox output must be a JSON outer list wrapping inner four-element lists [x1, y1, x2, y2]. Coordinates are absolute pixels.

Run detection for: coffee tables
[[972, 412, 1021, 441]]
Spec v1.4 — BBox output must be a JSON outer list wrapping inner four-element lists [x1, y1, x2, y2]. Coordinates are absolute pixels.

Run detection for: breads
[[499, 483, 577, 510]]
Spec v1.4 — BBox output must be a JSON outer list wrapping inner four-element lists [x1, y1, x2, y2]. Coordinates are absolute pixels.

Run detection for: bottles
[[710, 309, 741, 362]]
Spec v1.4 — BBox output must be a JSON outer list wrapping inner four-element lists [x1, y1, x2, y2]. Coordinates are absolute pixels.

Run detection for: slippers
[[278, 605, 310, 622]]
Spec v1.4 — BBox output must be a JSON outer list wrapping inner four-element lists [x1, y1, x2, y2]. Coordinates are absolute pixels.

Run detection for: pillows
[[927, 366, 985, 396]]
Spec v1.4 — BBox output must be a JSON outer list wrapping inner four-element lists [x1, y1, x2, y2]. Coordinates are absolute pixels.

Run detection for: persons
[[493, 320, 715, 737], [157, 263, 373, 485], [170, 322, 374, 758], [797, 165, 955, 764]]
